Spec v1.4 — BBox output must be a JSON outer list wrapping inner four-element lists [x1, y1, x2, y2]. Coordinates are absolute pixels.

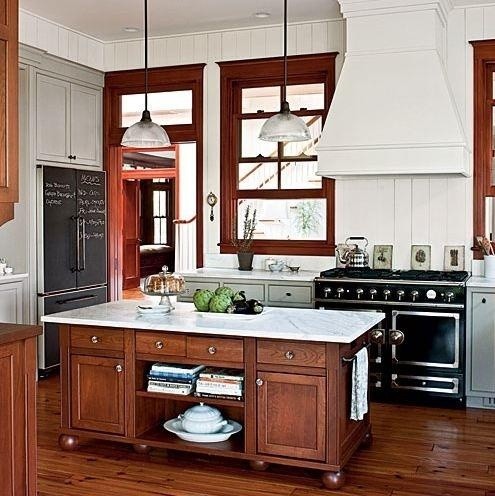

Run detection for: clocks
[[207, 192, 218, 221]]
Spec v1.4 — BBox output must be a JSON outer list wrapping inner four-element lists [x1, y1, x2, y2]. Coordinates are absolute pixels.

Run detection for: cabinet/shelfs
[[36, 72, 102, 166], [68, 325, 125, 436], [135, 333, 245, 460], [0, 282, 24, 325], [0, 0, 20, 204], [256, 340, 326, 462], [176, 280, 312, 308], [466, 287, 495, 399], [36, 166, 108, 296]]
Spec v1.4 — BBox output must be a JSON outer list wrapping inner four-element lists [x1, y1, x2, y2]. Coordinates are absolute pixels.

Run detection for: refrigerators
[[36, 164, 108, 378]]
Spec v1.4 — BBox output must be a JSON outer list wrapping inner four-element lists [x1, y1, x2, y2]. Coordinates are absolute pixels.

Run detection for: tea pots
[[336, 237, 369, 267]]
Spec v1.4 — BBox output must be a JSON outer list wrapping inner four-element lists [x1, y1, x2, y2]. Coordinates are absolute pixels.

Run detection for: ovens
[[314, 299, 466, 405]]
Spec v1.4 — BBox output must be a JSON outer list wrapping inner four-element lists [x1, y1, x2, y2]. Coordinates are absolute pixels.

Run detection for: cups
[[484, 255, 495, 279], [264, 257, 276, 271]]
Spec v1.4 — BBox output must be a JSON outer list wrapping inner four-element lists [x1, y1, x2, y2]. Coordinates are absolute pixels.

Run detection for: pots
[[178, 402, 227, 433]]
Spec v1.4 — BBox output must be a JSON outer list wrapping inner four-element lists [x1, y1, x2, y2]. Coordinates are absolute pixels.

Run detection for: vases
[[237, 252, 255, 271]]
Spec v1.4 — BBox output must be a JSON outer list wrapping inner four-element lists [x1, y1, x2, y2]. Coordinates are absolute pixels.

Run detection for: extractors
[[315, 0, 472, 180]]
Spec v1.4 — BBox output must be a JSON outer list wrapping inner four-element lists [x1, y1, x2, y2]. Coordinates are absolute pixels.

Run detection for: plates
[[191, 308, 271, 319], [136, 304, 172, 314]]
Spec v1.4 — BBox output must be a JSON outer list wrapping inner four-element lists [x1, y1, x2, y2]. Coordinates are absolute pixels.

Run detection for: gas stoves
[[315, 268, 472, 308]]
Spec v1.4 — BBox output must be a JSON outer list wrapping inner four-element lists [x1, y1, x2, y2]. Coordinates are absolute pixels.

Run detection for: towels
[[348, 346, 371, 421]]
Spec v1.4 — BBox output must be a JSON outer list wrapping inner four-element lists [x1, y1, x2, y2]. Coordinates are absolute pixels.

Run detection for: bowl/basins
[[289, 267, 300, 271], [269, 264, 283, 273], [164, 418, 243, 443]]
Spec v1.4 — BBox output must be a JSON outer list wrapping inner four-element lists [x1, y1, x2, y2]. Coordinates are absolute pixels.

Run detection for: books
[[146, 362, 245, 401]]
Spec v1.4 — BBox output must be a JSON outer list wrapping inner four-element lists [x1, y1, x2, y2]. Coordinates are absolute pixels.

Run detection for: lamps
[[120, 1, 172, 147], [258, 1, 311, 143]]
